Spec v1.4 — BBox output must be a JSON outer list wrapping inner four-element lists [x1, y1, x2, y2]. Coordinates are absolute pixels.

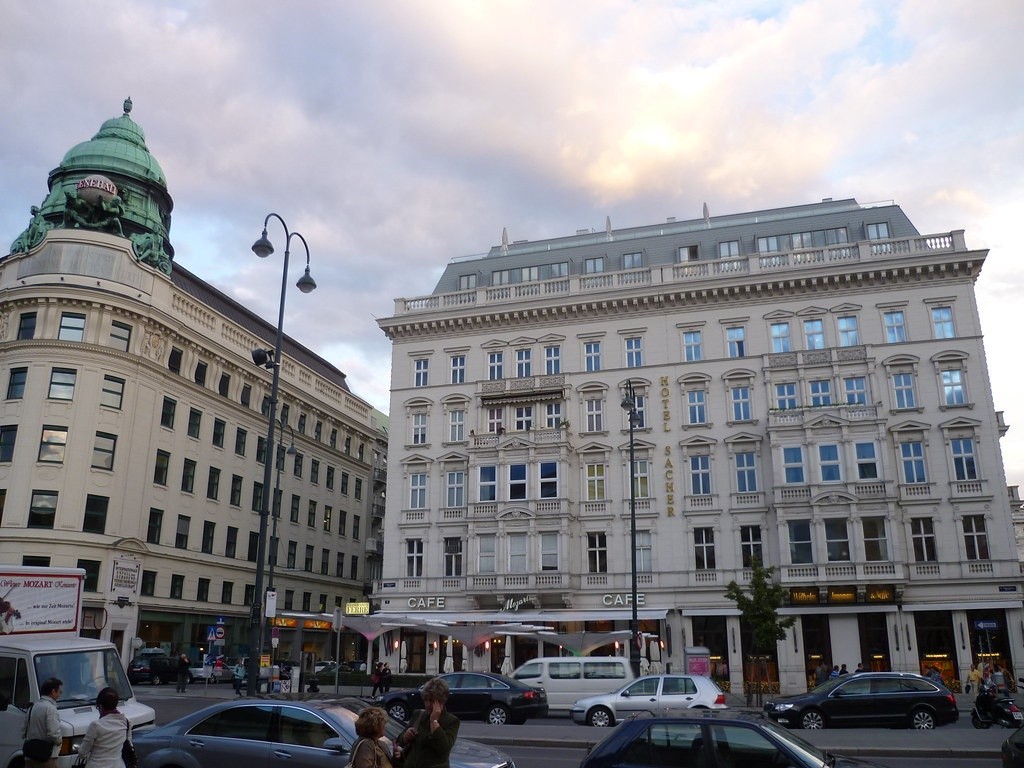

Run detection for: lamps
[[615, 641, 619, 651], [485, 641, 489, 651], [434, 640, 438, 651], [395, 640, 398, 650], [660, 640, 665, 651]]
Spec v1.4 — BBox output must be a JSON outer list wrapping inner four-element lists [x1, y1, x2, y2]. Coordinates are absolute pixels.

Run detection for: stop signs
[[216, 625, 227, 639]]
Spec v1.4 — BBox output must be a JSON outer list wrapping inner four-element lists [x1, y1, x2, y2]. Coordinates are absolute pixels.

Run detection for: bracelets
[[430, 719, 438, 723]]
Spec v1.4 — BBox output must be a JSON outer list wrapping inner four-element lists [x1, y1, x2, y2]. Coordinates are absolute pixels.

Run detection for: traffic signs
[[974, 622, 998, 629]]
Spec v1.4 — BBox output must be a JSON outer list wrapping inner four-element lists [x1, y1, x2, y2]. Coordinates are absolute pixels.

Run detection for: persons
[[78, 688, 132, 768], [349, 706, 401, 768], [19, 191, 166, 263], [280, 657, 393, 699], [21, 678, 63, 768], [397, 678, 460, 768], [814, 662, 864, 687], [233, 658, 245, 694], [925, 662, 1015, 703], [176, 654, 191, 693]]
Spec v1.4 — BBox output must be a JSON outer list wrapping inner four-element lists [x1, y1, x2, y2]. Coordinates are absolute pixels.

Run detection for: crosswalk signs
[[207, 626, 216, 641]]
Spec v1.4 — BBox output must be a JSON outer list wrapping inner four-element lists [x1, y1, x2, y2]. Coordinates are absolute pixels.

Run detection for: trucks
[[0, 566, 156, 768]]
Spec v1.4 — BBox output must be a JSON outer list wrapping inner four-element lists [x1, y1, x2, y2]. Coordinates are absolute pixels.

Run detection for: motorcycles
[[971, 677, 1023, 730]]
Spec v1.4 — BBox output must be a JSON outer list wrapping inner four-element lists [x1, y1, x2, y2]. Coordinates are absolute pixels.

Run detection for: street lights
[[243, 210, 317, 697], [620, 378, 642, 678]]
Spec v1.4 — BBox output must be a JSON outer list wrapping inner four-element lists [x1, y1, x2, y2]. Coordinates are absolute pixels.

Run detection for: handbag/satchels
[[72, 755, 87, 768], [371, 674, 380, 684], [122, 718, 138, 768], [22, 739, 56, 763]]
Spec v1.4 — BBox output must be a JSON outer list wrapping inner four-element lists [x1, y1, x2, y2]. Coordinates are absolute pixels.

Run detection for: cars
[[374, 671, 549, 726], [131, 693, 518, 768], [312, 660, 380, 673], [189, 660, 235, 683]]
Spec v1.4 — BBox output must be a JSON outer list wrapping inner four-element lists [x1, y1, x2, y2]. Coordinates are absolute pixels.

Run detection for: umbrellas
[[444, 635, 454, 674], [501, 636, 513, 676], [640, 633, 662, 676], [400, 640, 408, 673]]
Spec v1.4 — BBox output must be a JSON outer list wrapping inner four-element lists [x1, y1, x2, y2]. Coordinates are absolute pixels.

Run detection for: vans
[[510, 656, 636, 713]]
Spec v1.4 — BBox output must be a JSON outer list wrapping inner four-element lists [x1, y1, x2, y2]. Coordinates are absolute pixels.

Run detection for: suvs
[[763, 669, 960, 731], [576, 709, 888, 768], [128, 647, 194, 685], [571, 673, 731, 729]]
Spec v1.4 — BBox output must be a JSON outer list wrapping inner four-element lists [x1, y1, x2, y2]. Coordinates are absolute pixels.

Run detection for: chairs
[[689, 732, 705, 768]]
